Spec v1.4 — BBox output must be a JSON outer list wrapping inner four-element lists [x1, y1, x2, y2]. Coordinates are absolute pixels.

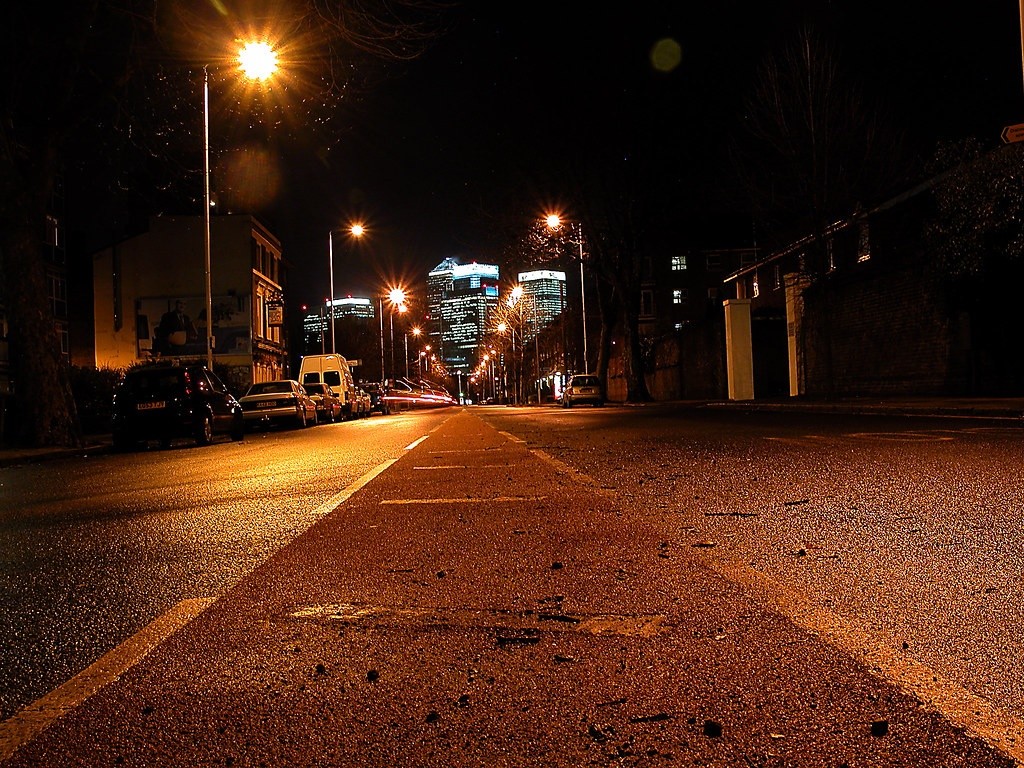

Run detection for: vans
[[297, 352, 355, 419]]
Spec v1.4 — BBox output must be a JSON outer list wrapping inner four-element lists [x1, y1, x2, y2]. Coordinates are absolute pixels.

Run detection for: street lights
[[484, 355, 496, 399], [548, 215, 588, 383], [379, 290, 403, 381], [330, 225, 363, 355], [390, 304, 407, 380], [405, 329, 420, 379], [202, 39, 276, 374], [513, 286, 541, 405]]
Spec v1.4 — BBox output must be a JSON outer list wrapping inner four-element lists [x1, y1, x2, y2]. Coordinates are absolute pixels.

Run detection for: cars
[[302, 382, 345, 423], [238, 380, 318, 433], [354, 385, 372, 417]]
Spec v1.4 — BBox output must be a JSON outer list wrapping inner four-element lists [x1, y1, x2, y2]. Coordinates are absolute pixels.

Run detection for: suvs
[[562, 374, 604, 409], [356, 381, 390, 416], [112, 365, 245, 454]]
